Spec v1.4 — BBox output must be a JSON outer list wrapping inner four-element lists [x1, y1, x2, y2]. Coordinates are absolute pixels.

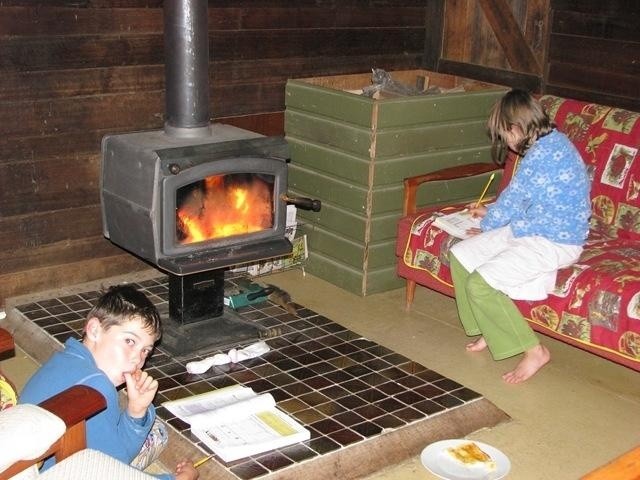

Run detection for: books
[[432, 208, 485, 240], [161, 382, 311, 465]]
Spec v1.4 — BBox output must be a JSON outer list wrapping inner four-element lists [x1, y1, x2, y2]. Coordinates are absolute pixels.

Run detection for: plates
[[418, 437, 511, 480]]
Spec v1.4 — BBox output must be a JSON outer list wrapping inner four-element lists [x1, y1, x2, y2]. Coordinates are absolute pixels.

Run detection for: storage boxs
[[285, 67, 513, 297]]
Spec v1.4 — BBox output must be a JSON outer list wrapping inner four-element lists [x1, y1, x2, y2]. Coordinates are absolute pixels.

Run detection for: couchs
[[398, 93, 640, 377]]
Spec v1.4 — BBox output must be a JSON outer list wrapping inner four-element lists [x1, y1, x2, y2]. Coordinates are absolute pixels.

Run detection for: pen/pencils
[[193, 453, 215, 468], [471, 173, 495, 217]]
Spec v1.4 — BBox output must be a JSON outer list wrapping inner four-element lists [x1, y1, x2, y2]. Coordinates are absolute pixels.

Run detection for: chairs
[[0, 330, 107, 480]]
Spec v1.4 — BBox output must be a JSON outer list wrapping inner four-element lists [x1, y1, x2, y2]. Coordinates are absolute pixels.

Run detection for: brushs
[[266, 284, 298, 315]]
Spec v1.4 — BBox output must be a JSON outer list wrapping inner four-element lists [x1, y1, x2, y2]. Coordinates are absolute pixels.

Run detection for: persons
[[448, 88, 592, 387], [15, 282, 202, 479]]
